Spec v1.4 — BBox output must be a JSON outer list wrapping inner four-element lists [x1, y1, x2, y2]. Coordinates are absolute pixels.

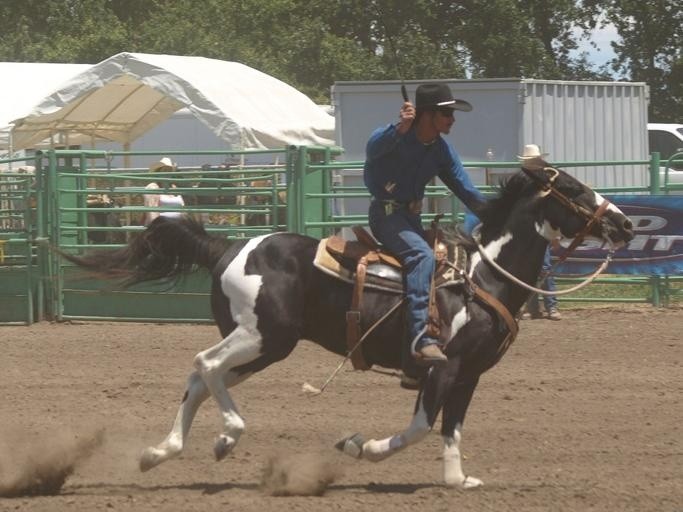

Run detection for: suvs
[[642, 119, 681, 197]]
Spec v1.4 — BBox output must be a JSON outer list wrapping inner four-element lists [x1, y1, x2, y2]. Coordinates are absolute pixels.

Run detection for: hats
[[149, 157, 178, 171], [516, 145, 550, 160], [415, 84, 473, 112]]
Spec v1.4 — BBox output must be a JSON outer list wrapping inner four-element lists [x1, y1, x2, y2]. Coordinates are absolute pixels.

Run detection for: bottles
[[485, 148, 493, 162]]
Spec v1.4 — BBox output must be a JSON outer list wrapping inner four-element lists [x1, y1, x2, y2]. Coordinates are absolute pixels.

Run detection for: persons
[[139, 157, 186, 228], [361, 82, 488, 368], [514, 142, 562, 322]]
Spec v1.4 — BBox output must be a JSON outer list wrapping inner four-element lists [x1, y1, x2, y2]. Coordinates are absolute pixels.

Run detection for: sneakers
[[420, 344, 449, 363], [522, 311, 543, 320], [549, 311, 561, 320]]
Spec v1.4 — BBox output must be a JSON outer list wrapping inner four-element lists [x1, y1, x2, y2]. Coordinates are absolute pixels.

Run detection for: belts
[[370, 193, 422, 212]]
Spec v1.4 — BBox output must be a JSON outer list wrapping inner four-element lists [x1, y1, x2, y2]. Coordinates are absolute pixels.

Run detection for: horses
[[46, 155, 636, 491]]
